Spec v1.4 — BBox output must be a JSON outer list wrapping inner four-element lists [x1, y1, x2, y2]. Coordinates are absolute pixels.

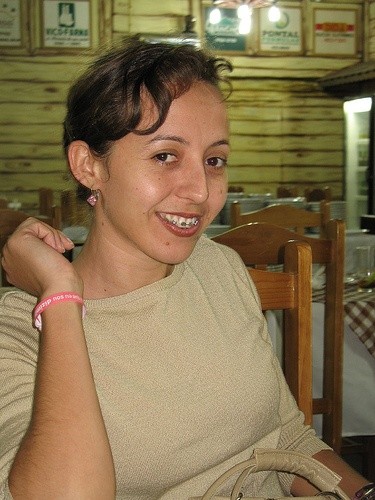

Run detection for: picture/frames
[[1, 1, 30, 55], [201, 5, 253, 55], [312, 8, 358, 57], [31, 1, 98, 55], [258, 6, 305, 54]]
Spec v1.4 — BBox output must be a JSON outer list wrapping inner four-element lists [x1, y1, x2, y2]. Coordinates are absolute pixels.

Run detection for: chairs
[[229, 200, 331, 241], [209, 220, 345, 455], [0, 188, 90, 288], [244, 241, 313, 429]]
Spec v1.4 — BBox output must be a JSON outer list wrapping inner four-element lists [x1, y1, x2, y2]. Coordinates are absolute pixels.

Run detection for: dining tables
[[265, 231, 374, 280], [266, 283, 375, 442], [223, 199, 344, 227]]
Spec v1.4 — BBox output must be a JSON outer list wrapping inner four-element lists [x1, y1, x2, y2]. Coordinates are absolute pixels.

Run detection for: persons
[[0, 45, 375, 500]]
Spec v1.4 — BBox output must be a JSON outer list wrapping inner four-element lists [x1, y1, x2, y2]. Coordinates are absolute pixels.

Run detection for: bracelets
[[355, 482, 375, 499], [32, 291, 87, 332]]
